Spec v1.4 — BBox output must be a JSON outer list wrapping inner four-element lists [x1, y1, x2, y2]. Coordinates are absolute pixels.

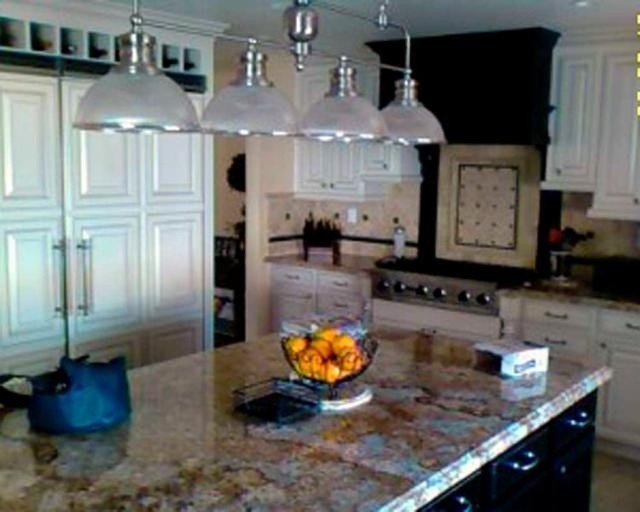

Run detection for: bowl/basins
[[281, 331, 379, 400]]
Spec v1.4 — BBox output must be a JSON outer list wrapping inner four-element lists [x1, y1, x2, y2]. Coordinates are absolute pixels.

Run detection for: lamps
[[70, 0, 203, 138], [376, 3, 450, 146], [300, 52, 395, 148], [198, 36, 309, 140]]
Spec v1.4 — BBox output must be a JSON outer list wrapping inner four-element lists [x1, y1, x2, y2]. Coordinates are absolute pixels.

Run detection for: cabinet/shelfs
[[0, 1, 231, 392], [263, 251, 374, 336], [534, 27, 640, 223], [493, 283, 640, 460], [290, 55, 424, 204]]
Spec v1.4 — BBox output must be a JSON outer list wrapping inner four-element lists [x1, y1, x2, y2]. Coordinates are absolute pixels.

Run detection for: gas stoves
[[375, 256, 533, 315]]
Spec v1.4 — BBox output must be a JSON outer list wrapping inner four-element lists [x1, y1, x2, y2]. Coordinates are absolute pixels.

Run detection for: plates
[[318, 385, 373, 412]]
